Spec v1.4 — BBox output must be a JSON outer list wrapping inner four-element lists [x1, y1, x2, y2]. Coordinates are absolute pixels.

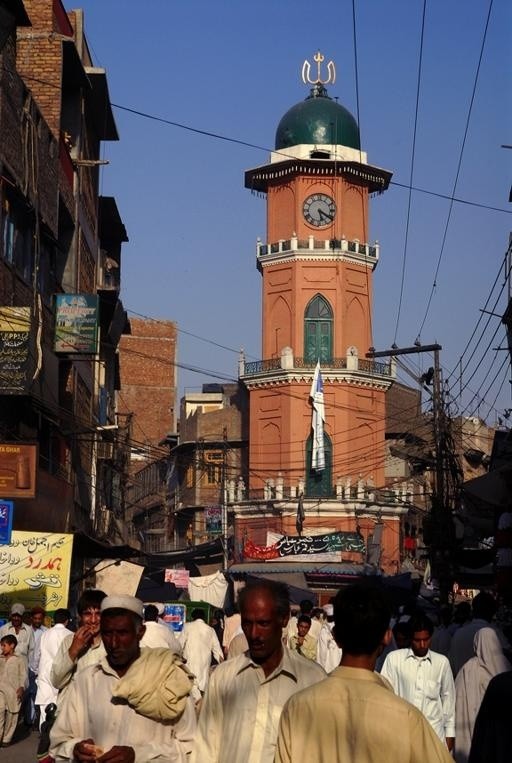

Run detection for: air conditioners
[[95, 509, 114, 535], [98, 441, 113, 459]]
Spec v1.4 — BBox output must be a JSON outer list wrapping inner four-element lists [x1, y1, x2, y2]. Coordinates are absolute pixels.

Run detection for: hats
[[11, 603, 27, 618], [99, 595, 145, 622]]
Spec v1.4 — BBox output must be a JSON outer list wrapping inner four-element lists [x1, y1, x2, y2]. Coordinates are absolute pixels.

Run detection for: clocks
[[303, 193, 338, 227]]
